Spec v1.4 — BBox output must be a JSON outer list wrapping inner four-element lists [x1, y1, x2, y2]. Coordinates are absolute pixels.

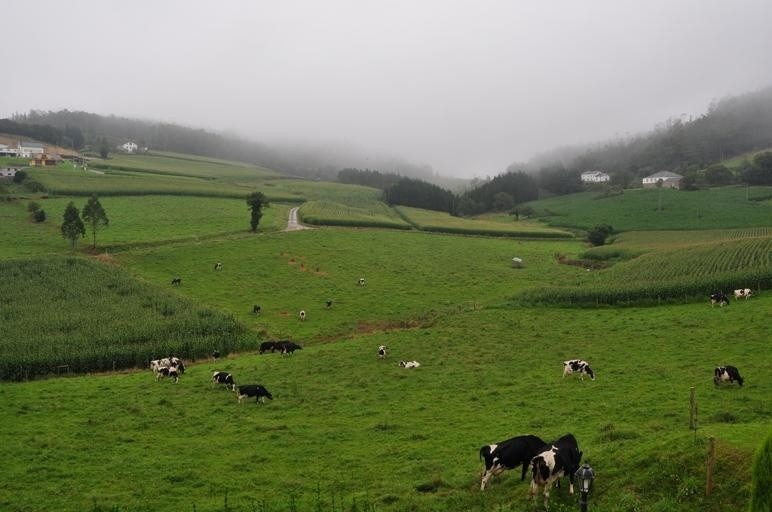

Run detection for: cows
[[258, 339, 303, 357], [398, 359, 421, 371], [562, 358, 595, 382], [377, 344, 387, 359], [715, 365, 744, 388], [479, 434, 553, 492], [212, 371, 236, 392], [708, 288, 755, 309], [235, 383, 273, 406], [531, 433, 583, 512], [148, 356, 188, 384]]
